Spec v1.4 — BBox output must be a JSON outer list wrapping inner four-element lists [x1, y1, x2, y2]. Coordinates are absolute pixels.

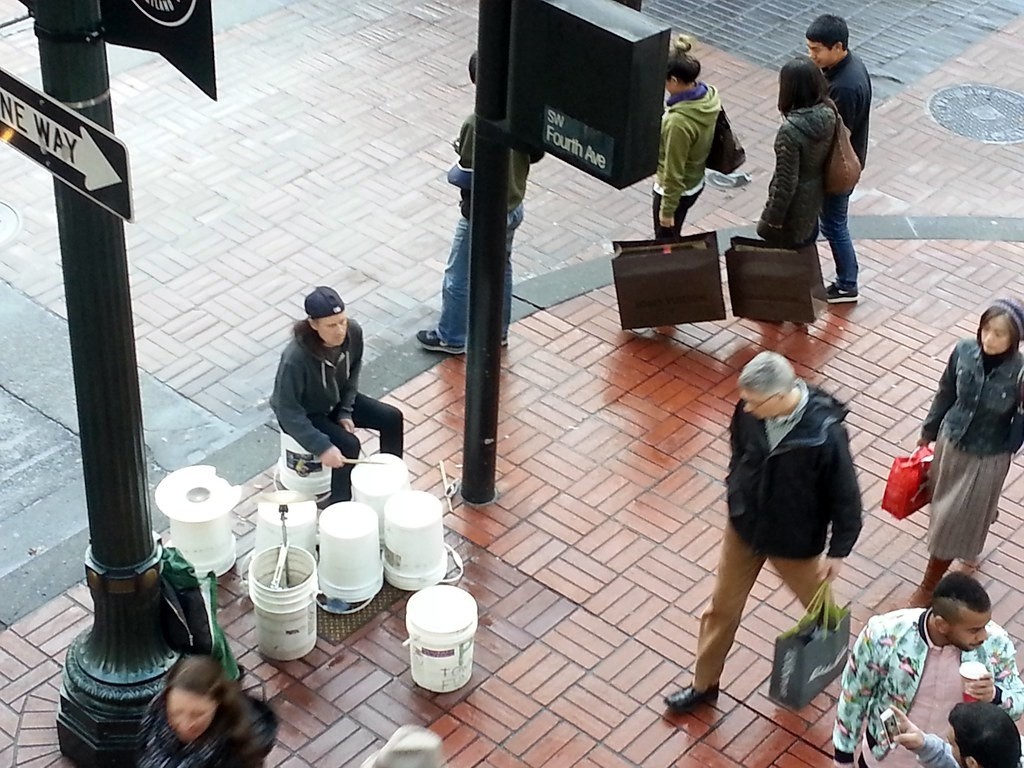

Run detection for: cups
[[959, 661, 986, 703]]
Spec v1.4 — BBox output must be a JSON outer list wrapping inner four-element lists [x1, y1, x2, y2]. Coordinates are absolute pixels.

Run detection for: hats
[[992, 297, 1024, 340], [304, 286, 345, 319]]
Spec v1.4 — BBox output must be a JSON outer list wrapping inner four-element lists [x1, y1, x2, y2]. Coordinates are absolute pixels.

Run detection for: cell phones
[[879, 708, 902, 750]]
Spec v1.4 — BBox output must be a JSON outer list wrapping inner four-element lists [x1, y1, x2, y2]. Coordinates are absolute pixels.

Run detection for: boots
[[903, 552, 954, 609]]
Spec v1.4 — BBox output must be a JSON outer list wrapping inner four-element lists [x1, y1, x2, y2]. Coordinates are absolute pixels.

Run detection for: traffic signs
[[0, 67, 134, 224]]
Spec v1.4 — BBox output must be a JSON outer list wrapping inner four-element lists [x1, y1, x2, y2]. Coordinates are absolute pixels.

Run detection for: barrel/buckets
[[349, 453, 412, 547], [246, 544, 318, 661], [253, 491, 319, 580], [314, 500, 385, 615], [170, 508, 237, 579], [404, 586, 479, 693], [380, 487, 463, 592], [272, 427, 336, 501]]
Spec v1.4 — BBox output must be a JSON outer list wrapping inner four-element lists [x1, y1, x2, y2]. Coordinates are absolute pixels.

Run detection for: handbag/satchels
[[611, 225, 726, 331], [881, 443, 934, 521], [769, 581, 851, 710], [706, 105, 746, 175], [826, 98, 861, 194], [159, 545, 212, 657], [723, 226, 827, 323]]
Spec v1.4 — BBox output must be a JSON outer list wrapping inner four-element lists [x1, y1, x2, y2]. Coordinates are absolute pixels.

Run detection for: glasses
[[738, 393, 781, 409]]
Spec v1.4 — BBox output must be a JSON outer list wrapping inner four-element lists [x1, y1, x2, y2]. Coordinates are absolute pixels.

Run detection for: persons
[[416, 48, 543, 355], [665, 351, 863, 707], [810, 13, 871, 302], [906, 297, 1024, 609], [757, 56, 838, 252], [133, 653, 277, 768], [271, 285, 403, 504], [650, 50, 721, 335], [833, 572, 1024, 768]]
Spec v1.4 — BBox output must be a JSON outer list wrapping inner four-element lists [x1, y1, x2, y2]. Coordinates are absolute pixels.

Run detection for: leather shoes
[[663, 683, 719, 708]]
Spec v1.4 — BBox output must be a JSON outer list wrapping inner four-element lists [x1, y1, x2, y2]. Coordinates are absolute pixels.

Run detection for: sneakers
[[416, 330, 465, 354], [825, 282, 858, 303]]
